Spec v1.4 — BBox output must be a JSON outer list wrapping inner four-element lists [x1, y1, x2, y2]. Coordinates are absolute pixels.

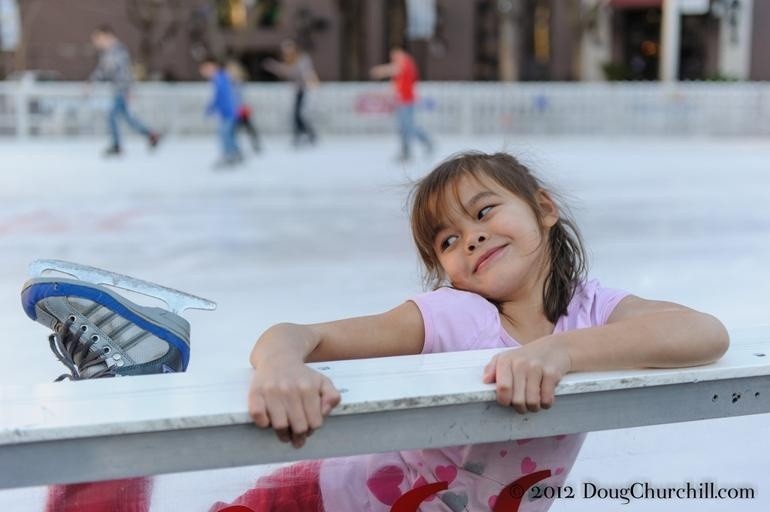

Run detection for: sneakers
[[21, 277, 191, 380]]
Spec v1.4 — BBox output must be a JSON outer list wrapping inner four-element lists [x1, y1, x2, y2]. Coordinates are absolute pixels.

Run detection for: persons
[[222, 60, 264, 157], [19, 149, 733, 511], [84, 25, 166, 161], [198, 55, 241, 163], [260, 31, 321, 148], [367, 42, 434, 164]]
